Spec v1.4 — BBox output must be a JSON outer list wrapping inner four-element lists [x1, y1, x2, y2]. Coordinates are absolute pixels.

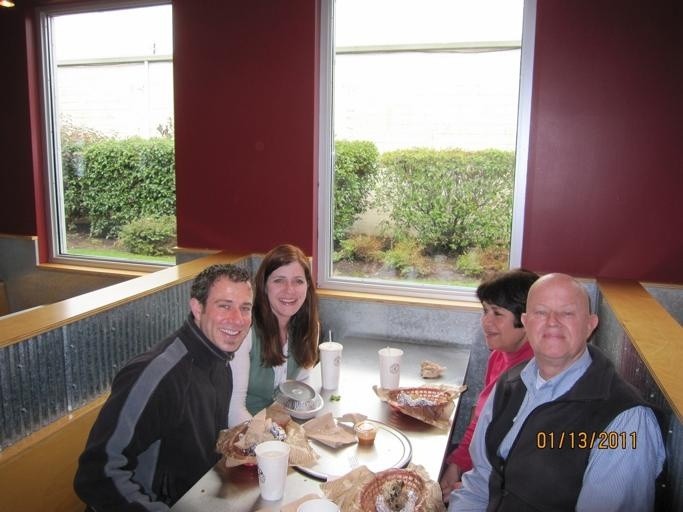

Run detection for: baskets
[[387, 388, 453, 417], [359, 470, 428, 512]]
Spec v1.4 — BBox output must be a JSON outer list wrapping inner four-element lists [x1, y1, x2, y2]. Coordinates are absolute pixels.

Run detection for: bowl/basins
[[276, 391, 325, 420]]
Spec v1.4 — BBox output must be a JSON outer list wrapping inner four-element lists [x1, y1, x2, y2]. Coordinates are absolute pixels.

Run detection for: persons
[[226, 242, 319, 429], [69, 263, 255, 511], [444, 273, 668, 511], [435, 267, 540, 506]]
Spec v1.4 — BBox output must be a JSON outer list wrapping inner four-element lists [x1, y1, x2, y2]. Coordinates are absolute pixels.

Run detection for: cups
[[354, 421, 378, 447], [254, 441, 288, 503], [319, 341, 344, 391], [376, 348, 402, 389]]
